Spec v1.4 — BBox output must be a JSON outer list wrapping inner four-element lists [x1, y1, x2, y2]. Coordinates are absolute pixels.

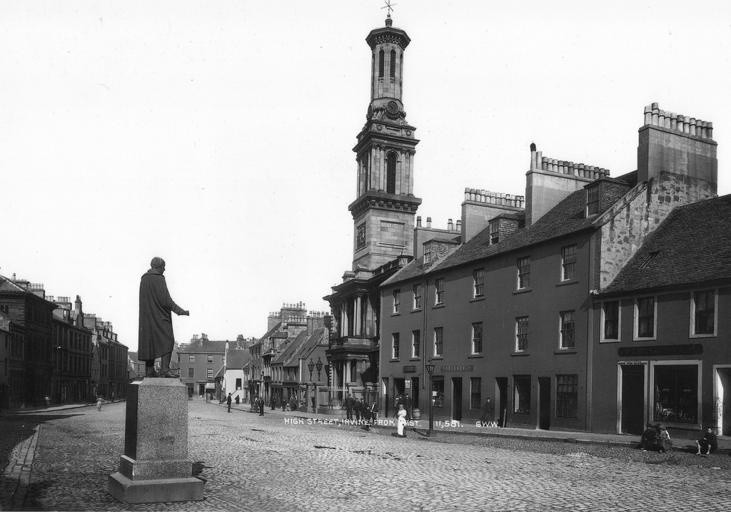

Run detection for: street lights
[[425, 360, 436, 436]]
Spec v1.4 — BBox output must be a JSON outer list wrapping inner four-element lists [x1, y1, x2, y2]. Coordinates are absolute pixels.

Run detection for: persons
[[696, 427, 717, 456], [228, 393, 232, 413], [656, 424, 672, 453], [394, 393, 411, 436], [259, 398, 264, 416], [138, 257, 189, 378], [485, 398, 493, 416], [343, 394, 379, 426], [640, 424, 658, 451]]
